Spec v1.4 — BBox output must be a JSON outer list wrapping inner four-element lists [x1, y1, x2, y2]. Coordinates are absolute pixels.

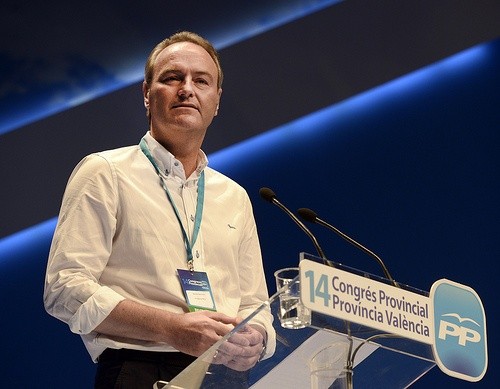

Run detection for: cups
[[274, 268, 313, 328]]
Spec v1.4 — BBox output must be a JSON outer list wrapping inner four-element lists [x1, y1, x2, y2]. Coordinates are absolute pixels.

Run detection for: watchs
[[257, 339, 267, 361]]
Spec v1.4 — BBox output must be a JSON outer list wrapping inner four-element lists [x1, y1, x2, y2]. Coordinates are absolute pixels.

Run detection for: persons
[[43, 32, 276, 389]]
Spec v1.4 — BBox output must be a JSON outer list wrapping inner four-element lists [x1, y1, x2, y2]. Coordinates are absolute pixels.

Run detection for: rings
[[214, 350, 219, 359]]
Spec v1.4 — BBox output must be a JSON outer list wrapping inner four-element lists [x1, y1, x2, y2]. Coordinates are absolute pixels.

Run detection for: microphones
[[259, 187, 353, 389], [297, 208, 398, 389]]
